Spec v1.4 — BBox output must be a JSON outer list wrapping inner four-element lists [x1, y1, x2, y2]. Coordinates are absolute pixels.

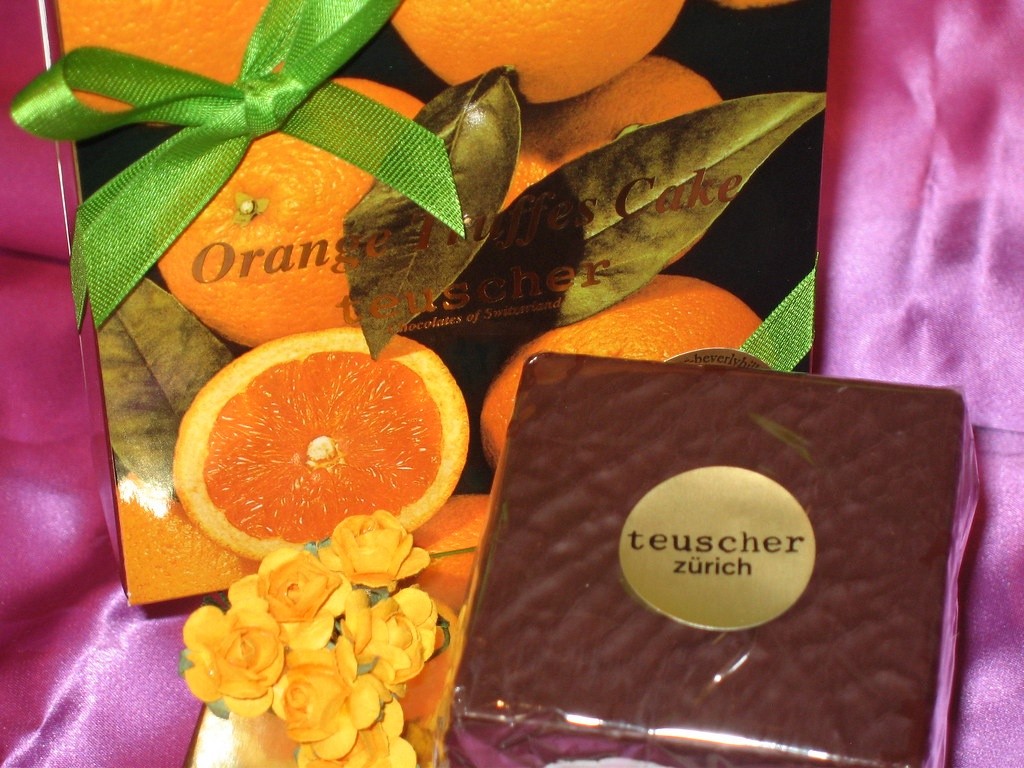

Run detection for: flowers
[[182, 507, 437, 768]]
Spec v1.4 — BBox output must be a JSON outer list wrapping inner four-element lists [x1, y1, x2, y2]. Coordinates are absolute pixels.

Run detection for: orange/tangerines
[[56, 0, 771, 727]]
